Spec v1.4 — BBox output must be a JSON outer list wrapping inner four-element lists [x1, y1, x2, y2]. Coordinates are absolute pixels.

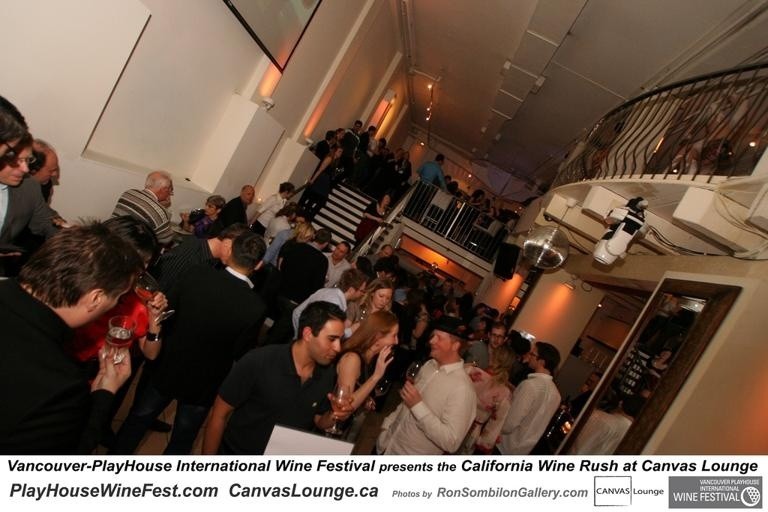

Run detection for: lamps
[[261, 95, 276, 114], [563, 275, 579, 292], [304, 136, 314, 148]]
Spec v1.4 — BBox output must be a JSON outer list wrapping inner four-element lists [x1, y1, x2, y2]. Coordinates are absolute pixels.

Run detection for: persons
[[563, 397, 641, 456], [0, 218, 144, 454], [316, 311, 401, 421], [97, 223, 252, 440], [200, 300, 355, 455], [298, 121, 412, 222], [467, 304, 514, 367], [252, 181, 398, 345], [0, 132, 60, 276], [71, 214, 170, 420], [569, 371, 602, 418], [0, 97, 28, 157], [406, 153, 517, 265], [495, 342, 562, 454], [376, 315, 478, 454], [105, 233, 268, 455], [22, 139, 58, 202], [631, 349, 673, 395], [219, 186, 255, 234], [111, 172, 175, 243], [456, 347, 513, 454], [394, 270, 469, 354], [182, 196, 225, 232]]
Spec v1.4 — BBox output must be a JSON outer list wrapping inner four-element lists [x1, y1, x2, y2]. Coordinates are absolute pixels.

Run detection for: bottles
[[560, 418, 573, 433], [544, 409, 565, 441], [564, 418, 574, 434]]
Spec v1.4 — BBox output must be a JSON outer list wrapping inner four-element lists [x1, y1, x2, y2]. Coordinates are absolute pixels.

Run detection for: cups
[[325, 274, 329, 284], [333, 282, 340, 288], [100, 315, 136, 364]]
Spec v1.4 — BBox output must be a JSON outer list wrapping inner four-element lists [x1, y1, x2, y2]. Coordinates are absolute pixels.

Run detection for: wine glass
[[372, 378, 391, 401], [358, 306, 370, 322], [471, 351, 479, 367], [406, 362, 424, 386], [325, 385, 349, 434], [133, 270, 175, 326]]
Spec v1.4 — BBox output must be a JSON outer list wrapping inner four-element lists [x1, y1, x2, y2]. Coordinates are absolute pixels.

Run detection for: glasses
[[491, 333, 505, 339], [9, 157, 37, 167], [5, 143, 15, 157]]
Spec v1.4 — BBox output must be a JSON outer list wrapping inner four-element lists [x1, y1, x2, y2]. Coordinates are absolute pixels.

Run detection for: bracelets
[[147, 331, 161, 343]]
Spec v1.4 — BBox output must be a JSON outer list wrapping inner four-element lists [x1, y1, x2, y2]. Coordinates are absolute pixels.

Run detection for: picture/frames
[[223, 0, 323, 74]]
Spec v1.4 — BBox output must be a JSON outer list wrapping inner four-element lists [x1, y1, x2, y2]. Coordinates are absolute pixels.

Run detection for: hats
[[431, 315, 471, 339]]
